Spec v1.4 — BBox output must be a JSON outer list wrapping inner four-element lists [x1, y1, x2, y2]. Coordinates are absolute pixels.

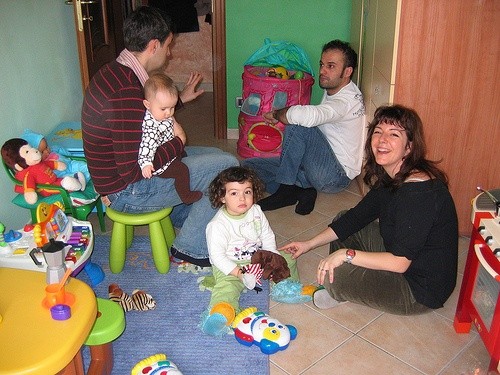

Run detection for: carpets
[[76, 234, 270, 375]]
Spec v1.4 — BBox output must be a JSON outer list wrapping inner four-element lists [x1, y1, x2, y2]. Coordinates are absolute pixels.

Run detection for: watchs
[[344, 249, 356, 264]]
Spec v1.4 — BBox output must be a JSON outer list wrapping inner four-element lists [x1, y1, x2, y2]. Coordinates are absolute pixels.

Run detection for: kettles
[[30, 238, 73, 286]]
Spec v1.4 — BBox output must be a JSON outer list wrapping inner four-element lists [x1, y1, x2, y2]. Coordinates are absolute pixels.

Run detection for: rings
[[318, 268, 327, 272]]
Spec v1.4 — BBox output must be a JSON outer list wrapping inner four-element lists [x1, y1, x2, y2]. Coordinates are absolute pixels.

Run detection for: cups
[[45, 284, 64, 306]]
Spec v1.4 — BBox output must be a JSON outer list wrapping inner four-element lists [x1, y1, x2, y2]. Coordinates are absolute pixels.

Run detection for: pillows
[[11, 180, 100, 209]]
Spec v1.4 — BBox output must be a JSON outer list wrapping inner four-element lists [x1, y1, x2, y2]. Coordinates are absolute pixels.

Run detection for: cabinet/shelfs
[[350, 0, 500, 238]]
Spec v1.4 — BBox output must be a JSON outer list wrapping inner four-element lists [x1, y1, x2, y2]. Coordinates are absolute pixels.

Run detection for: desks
[[0, 268, 98, 375]]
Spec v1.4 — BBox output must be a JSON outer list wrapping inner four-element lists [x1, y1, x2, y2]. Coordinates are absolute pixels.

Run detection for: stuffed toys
[[109, 282, 157, 313], [1, 128, 91, 204], [251, 250, 291, 284]]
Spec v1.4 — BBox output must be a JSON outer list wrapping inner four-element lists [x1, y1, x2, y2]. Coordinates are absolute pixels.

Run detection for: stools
[[106, 206, 177, 274], [83, 296, 126, 375]]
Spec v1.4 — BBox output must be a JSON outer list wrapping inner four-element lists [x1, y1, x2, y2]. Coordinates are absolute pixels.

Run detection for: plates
[[43, 292, 75, 309]]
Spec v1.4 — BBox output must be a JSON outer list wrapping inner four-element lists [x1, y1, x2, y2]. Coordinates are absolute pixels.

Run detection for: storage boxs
[[48, 120, 105, 213]]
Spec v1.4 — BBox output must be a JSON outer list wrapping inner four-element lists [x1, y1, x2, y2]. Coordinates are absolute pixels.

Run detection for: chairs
[[1, 152, 107, 234]]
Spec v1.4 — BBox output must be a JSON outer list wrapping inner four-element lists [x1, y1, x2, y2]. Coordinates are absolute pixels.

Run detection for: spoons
[[56, 268, 72, 291]]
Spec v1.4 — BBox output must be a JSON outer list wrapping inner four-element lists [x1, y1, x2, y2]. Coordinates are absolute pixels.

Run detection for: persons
[[81, 6, 239, 267], [137, 73, 203, 205], [276, 105, 459, 316], [239, 39, 366, 215], [200, 166, 317, 336]]
